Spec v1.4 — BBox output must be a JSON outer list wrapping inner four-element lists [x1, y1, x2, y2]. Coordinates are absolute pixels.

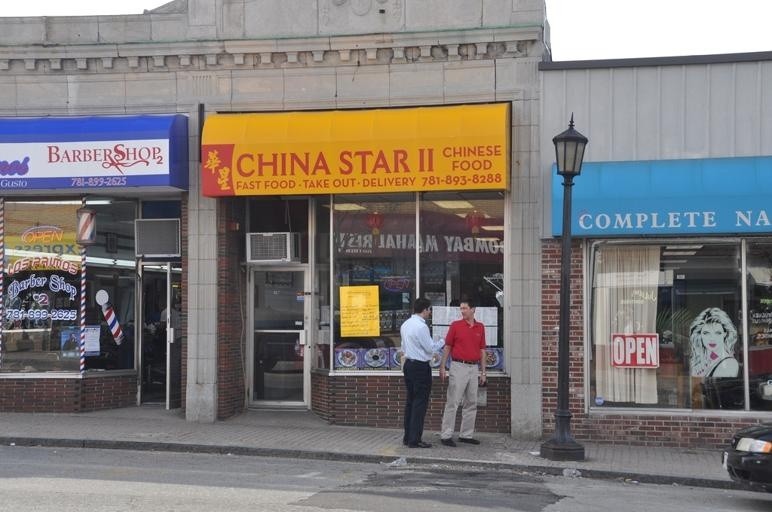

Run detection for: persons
[[157, 298, 181, 341], [440, 301, 486, 447], [63, 333, 80, 351], [400, 298, 446, 448], [688, 307, 739, 378]]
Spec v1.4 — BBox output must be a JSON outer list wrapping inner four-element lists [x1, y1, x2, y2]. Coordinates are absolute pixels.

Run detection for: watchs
[[479, 367, 486, 375]]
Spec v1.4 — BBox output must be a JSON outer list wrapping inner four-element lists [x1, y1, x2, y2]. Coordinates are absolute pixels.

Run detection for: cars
[[721, 420, 772, 494]]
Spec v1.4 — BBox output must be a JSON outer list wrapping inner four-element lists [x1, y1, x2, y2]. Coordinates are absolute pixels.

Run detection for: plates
[[392, 350, 401, 365], [337, 350, 358, 368], [430, 352, 442, 368], [480, 351, 499, 368], [363, 348, 387, 369]]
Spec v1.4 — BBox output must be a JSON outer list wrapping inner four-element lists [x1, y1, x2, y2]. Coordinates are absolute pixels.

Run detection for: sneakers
[[440, 438, 457, 447], [403, 439, 433, 448], [458, 438, 481, 445]]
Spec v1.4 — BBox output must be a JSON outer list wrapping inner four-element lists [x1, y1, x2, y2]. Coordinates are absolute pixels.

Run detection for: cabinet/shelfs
[[736, 261, 772, 401]]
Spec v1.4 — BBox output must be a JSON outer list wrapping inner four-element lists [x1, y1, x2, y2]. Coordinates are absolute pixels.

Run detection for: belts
[[451, 358, 479, 365], [408, 359, 430, 365]]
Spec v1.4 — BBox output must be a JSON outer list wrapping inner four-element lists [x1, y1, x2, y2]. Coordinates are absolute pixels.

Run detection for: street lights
[[541, 112, 588, 463]]
